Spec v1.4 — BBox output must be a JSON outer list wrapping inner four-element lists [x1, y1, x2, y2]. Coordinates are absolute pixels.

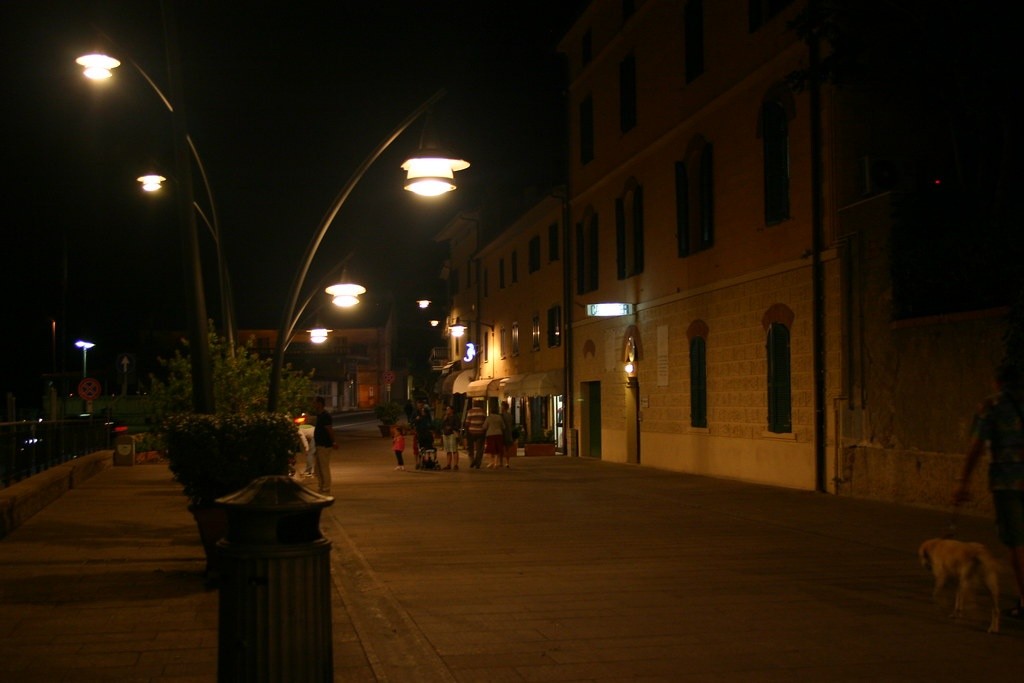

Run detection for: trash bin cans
[[114, 435, 136, 466], [215, 473, 337, 683]]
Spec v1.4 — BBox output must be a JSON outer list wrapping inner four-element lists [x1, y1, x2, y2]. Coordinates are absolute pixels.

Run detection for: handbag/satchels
[[512, 429, 519, 439]]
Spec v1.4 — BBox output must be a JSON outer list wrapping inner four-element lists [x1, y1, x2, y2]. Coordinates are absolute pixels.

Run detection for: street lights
[[136, 168, 238, 346], [74, 339, 97, 378], [261, 86, 472, 414], [71, 20, 240, 415], [284, 308, 333, 353], [283, 255, 368, 343]]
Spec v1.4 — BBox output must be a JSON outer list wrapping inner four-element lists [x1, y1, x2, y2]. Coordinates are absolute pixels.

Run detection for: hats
[[423, 404, 430, 412]]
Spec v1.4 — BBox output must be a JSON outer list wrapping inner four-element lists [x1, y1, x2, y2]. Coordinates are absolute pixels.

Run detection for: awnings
[[499, 370, 565, 399], [443, 369, 475, 394], [467, 377, 509, 398]]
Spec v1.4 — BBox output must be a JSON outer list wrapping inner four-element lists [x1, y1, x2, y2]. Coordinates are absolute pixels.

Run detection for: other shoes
[[470, 460, 476, 468], [394, 466, 405, 471], [487, 464, 494, 468], [1001, 599, 1024, 619], [454, 465, 458, 471], [442, 465, 451, 470], [499, 465, 504, 468], [506, 464, 510, 468]]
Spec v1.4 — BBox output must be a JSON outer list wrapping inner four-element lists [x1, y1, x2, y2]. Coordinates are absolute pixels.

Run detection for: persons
[[410, 398, 431, 470], [295, 425, 318, 475], [439, 405, 461, 471], [482, 406, 506, 469], [498, 401, 512, 468], [464, 401, 487, 469], [311, 396, 336, 495], [390, 427, 405, 471], [950, 365, 1024, 621]]
